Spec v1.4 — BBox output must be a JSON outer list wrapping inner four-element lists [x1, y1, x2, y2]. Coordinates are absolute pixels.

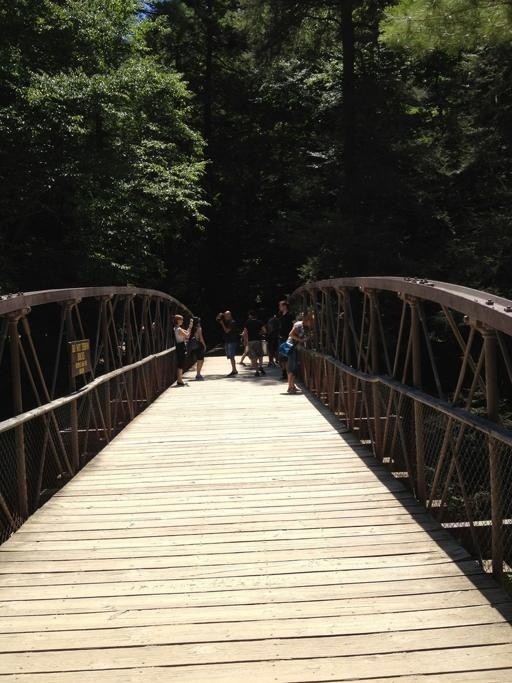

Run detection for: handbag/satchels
[[190, 337, 204, 353], [277, 342, 296, 356]]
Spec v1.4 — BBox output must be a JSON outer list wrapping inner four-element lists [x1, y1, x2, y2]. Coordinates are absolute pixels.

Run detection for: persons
[[279, 315, 315, 394], [238, 300, 295, 377], [216, 310, 239, 376], [172, 314, 193, 386], [189, 318, 206, 380]]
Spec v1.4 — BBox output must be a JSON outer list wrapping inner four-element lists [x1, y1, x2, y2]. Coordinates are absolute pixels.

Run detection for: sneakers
[[228, 371, 238, 376], [196, 375, 205, 381], [177, 381, 188, 385], [268, 361, 281, 368], [287, 386, 301, 394], [256, 367, 265, 377]]
